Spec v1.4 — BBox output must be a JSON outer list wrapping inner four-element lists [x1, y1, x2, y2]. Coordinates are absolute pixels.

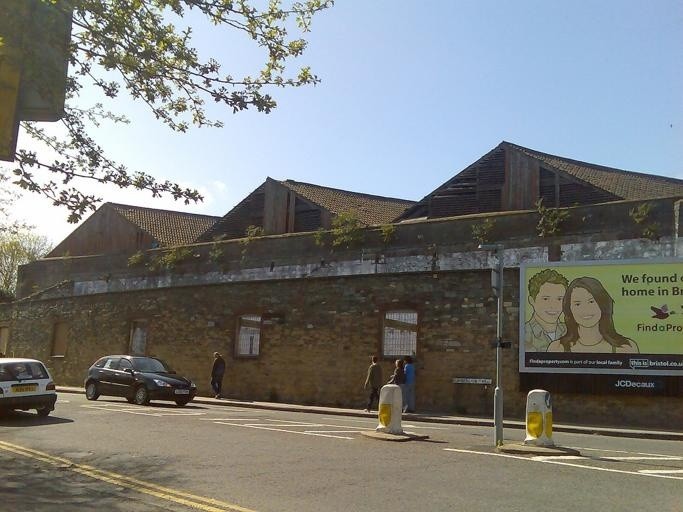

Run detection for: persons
[[524, 269, 640, 353], [210, 352, 225, 399], [363, 356, 417, 412]]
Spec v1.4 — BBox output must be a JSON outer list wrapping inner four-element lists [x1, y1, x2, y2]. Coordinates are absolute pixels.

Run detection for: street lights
[[477, 244, 504, 447]]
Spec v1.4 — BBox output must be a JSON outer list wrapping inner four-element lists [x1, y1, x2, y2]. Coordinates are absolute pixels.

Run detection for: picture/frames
[[517, 256, 683, 377]]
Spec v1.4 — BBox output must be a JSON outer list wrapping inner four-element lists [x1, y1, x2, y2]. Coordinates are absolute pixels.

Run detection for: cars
[[85, 354, 199, 406], [0, 357, 57, 416]]
